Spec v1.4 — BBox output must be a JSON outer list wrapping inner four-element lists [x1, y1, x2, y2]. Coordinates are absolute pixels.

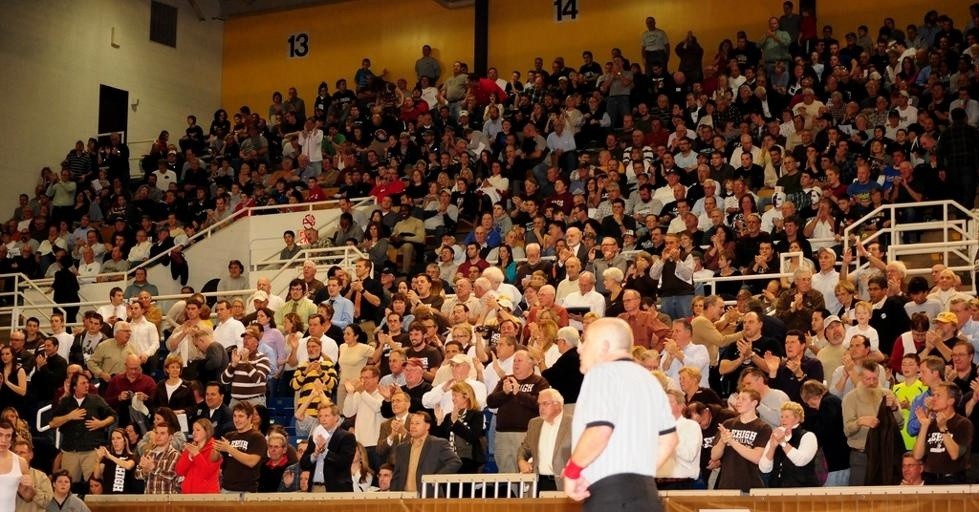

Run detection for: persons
[[561, 316, 679, 511], [45, 470, 93, 512], [1, 3, 978, 495], [0, 420, 33, 512], [12, 439, 55, 512]]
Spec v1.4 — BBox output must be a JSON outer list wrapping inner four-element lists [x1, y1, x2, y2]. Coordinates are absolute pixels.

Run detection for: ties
[[353, 279, 363, 318], [292, 302, 298, 312], [329, 299, 335, 309], [113, 308, 118, 316]]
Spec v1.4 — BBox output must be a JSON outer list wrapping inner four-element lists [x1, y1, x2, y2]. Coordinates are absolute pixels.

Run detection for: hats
[[818, 246, 836, 260], [241, 328, 260, 340], [497, 299, 513, 311], [823, 315, 843, 328], [448, 353, 471, 368], [933, 312, 959, 324], [621, 229, 636, 238], [402, 357, 423, 369], [252, 289, 269, 302]]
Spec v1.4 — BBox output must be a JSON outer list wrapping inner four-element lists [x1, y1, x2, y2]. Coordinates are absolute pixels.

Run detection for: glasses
[[11, 338, 24, 342], [292, 287, 300, 292], [88, 320, 99, 326], [624, 297, 635, 303]]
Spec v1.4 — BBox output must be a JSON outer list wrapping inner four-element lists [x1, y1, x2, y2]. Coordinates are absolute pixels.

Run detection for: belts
[[67, 447, 94, 452], [656, 476, 683, 483], [312, 483, 325, 486], [539, 474, 554, 481]]
